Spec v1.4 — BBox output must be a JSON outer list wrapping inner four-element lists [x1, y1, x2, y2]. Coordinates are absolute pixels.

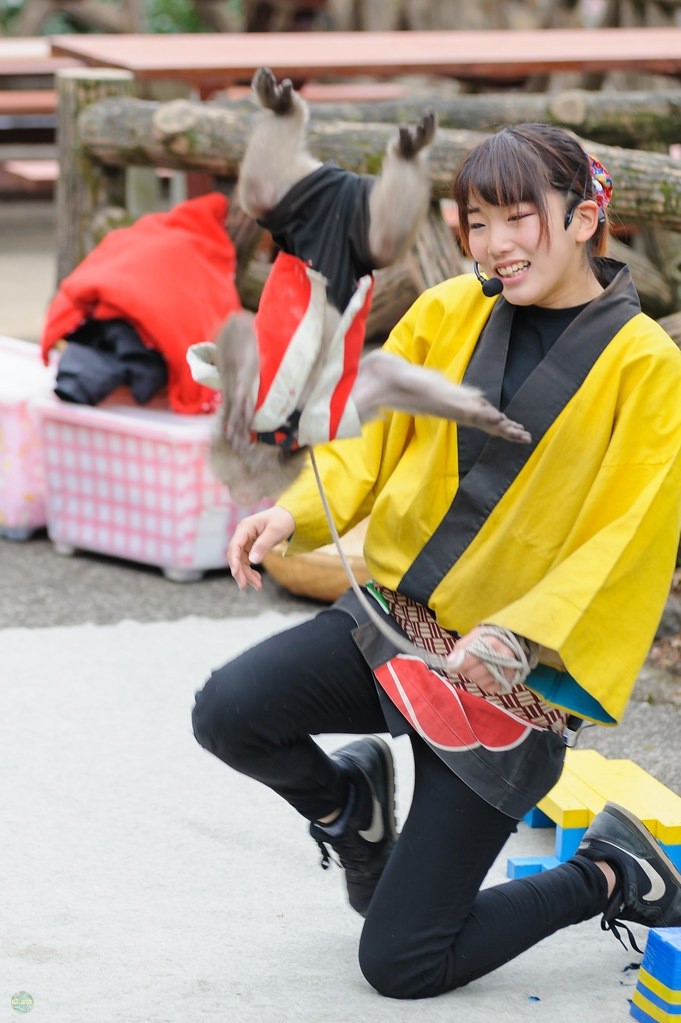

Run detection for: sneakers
[[576, 800, 681, 927], [311, 736, 397, 919]]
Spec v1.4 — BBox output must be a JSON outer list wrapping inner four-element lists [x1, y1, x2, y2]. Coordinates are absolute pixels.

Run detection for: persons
[[189, 121, 680, 1004]]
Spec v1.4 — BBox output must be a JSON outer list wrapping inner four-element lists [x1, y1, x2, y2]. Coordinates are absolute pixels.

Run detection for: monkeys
[[208, 66, 535, 507]]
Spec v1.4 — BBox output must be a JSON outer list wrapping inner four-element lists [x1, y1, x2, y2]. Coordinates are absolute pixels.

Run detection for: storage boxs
[[0, 334, 276, 592]]
